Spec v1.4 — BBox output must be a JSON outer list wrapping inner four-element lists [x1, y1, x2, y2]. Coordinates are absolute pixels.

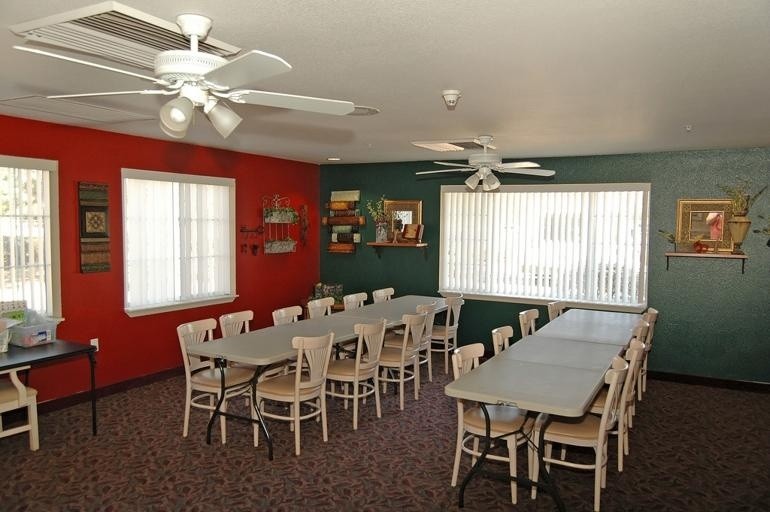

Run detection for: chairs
[[448, 300, 660, 510], [177, 287, 465, 461], [0, 362, 43, 458]]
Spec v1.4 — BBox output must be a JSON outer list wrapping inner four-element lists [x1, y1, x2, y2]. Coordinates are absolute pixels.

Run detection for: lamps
[[157, 86, 244, 139], [461, 169, 504, 193]]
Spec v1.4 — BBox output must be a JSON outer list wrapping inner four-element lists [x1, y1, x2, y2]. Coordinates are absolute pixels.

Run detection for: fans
[[413, 137, 559, 177], [8, 14, 355, 118]]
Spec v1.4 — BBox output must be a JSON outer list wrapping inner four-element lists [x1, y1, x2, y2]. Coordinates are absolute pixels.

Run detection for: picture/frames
[[675, 198, 735, 252], [382, 199, 422, 244]]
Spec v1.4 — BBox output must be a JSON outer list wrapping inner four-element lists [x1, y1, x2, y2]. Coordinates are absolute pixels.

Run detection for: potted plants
[[722, 176, 766, 255], [368, 196, 394, 244]]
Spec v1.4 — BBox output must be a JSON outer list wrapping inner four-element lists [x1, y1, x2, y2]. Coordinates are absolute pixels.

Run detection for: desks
[[0, 338, 98, 440]]
